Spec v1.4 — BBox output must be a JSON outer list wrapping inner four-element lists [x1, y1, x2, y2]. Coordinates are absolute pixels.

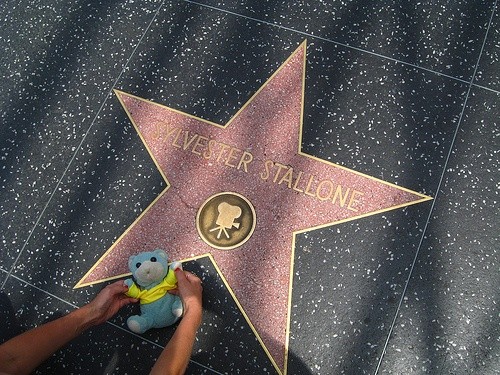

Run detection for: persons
[[0, 267, 204, 375]]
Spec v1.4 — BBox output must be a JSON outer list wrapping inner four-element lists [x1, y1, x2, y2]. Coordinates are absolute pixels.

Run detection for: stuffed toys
[[120, 248, 188, 335]]
